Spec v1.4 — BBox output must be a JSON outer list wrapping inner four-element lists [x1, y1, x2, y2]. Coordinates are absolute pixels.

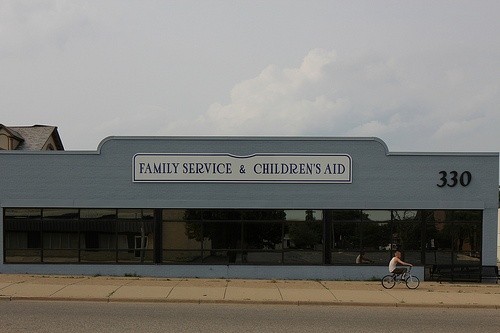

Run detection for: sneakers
[[399, 279, 408, 284]]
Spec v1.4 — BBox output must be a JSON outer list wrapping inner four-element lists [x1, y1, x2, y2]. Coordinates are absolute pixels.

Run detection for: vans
[[379, 243, 398, 252]]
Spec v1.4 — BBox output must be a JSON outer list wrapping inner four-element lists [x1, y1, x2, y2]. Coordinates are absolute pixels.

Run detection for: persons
[[355, 251, 375, 264], [388, 250, 413, 282]]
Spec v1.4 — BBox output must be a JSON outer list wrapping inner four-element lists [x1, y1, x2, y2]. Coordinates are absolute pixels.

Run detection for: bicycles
[[381, 265, 420, 289]]
